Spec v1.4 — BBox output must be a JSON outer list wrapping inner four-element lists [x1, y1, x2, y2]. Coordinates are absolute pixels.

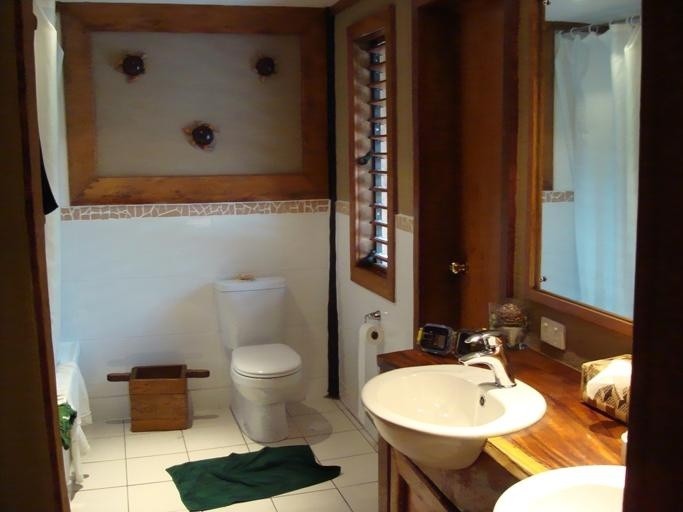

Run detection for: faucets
[[458, 329, 518, 387]]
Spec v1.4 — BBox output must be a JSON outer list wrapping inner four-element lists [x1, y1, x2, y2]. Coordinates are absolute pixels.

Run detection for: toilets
[[215, 275, 304, 443]]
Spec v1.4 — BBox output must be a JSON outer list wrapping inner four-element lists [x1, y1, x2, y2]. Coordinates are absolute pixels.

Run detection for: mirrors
[[524, 0, 645, 338]]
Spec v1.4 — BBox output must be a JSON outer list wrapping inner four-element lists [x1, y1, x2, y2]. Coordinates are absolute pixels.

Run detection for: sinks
[[492, 465, 626, 512], [359, 365, 547, 472]]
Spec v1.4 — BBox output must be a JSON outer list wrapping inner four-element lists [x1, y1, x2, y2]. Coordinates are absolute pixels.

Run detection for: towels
[[163, 442, 342, 512]]
